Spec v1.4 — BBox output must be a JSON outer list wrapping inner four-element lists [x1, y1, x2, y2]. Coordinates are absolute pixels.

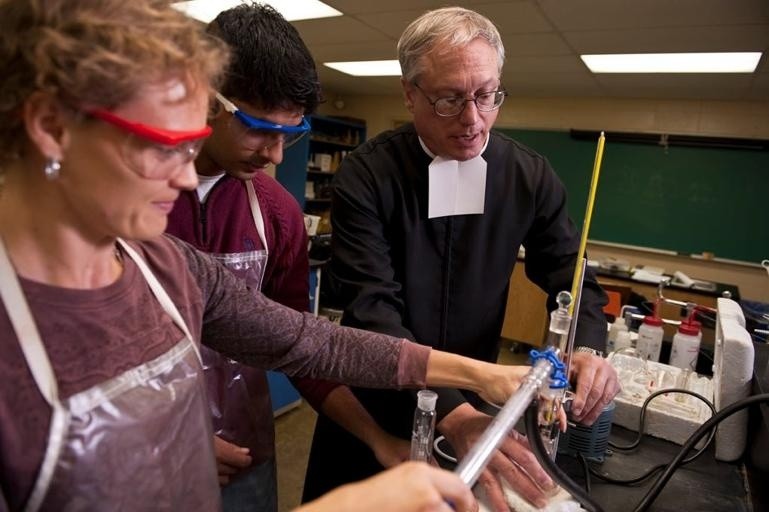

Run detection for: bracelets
[[574, 345, 605, 359]]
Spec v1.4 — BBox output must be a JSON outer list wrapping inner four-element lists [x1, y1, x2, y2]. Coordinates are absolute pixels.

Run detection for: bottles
[[607, 299, 703, 418], [537, 291, 573, 463], [454, 345, 562, 490], [408, 390, 439, 464]]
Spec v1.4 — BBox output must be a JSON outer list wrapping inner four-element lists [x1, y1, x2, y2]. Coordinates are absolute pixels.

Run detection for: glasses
[[69, 101, 212, 181], [411, 80, 509, 118], [209, 87, 312, 156]]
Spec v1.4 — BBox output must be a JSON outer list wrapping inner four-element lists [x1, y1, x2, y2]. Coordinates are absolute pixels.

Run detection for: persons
[[161, 1, 442, 511], [300, 0, 620, 511], [1, 1, 569, 511]]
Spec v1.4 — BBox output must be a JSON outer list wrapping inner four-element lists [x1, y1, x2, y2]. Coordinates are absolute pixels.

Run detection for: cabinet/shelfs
[[264, 261, 321, 419], [272, 113, 368, 264]]
